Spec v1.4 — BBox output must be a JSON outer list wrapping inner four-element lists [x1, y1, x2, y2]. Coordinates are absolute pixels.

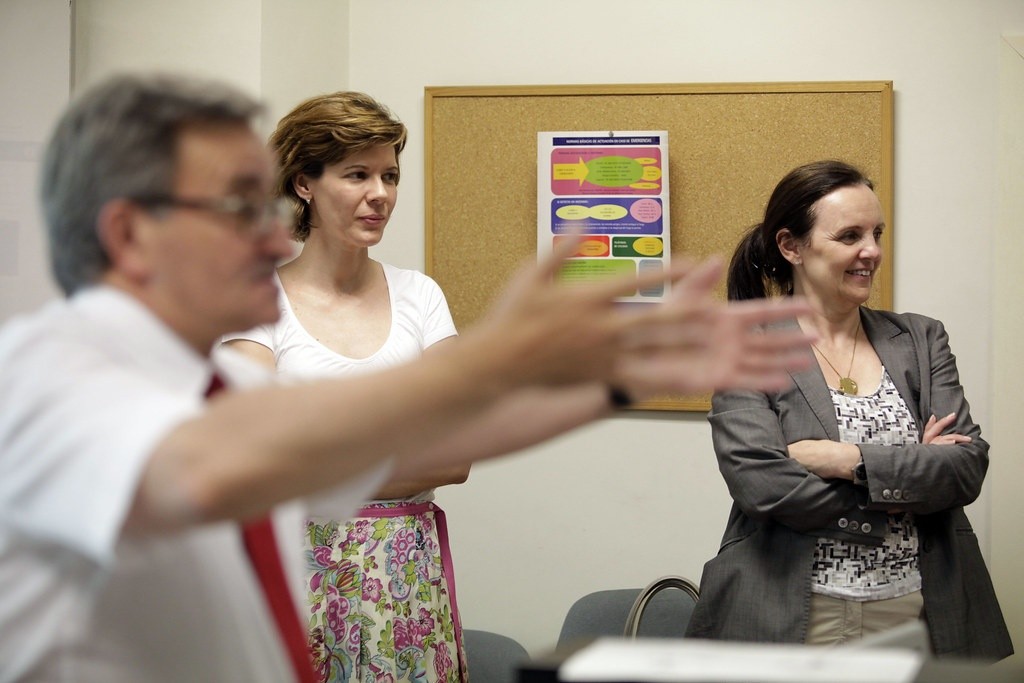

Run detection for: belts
[[355, 501, 464, 683]]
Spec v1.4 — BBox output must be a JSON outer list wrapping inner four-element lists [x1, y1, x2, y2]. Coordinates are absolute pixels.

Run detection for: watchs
[[851, 454, 867, 485]]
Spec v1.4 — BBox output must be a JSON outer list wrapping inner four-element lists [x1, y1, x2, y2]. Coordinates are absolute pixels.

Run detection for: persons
[[682, 160, 1015, 666], [214, 92, 470, 683], [0, 63, 819, 683]]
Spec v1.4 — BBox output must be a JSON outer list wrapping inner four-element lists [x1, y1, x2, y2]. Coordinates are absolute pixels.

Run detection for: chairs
[[556, 588, 696, 649], [462, 629, 531, 683]]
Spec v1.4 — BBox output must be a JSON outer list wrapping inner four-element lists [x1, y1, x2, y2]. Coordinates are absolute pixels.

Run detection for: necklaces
[[811, 319, 861, 395]]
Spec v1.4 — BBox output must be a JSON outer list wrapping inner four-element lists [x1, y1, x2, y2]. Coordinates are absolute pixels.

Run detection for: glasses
[[133, 196, 285, 231]]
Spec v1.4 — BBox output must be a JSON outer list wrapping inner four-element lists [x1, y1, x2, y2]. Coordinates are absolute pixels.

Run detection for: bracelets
[[607, 385, 633, 410]]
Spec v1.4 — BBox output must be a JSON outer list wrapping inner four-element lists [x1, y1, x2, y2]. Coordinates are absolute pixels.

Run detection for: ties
[[204, 371, 319, 683]]
